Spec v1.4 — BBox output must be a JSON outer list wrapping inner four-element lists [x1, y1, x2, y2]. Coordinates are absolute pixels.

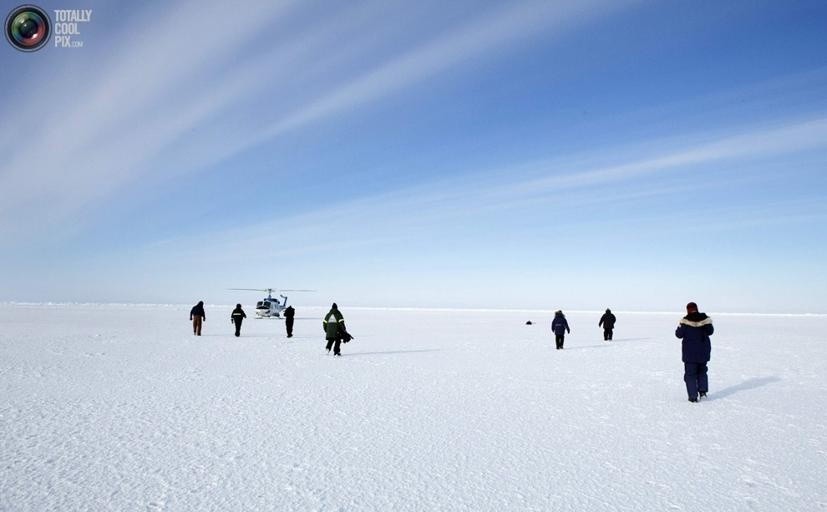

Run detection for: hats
[[686, 302, 698, 313]]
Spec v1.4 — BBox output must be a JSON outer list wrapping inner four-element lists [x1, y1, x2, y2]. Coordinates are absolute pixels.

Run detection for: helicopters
[[221, 284, 318, 320]]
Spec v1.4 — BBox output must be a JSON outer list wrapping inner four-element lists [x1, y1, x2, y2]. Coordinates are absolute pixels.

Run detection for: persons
[[599, 308, 616, 340], [675, 301, 714, 403], [552, 310, 571, 349], [323, 303, 346, 356], [284, 305, 295, 338], [189, 302, 205, 337], [230, 303, 247, 337]]
